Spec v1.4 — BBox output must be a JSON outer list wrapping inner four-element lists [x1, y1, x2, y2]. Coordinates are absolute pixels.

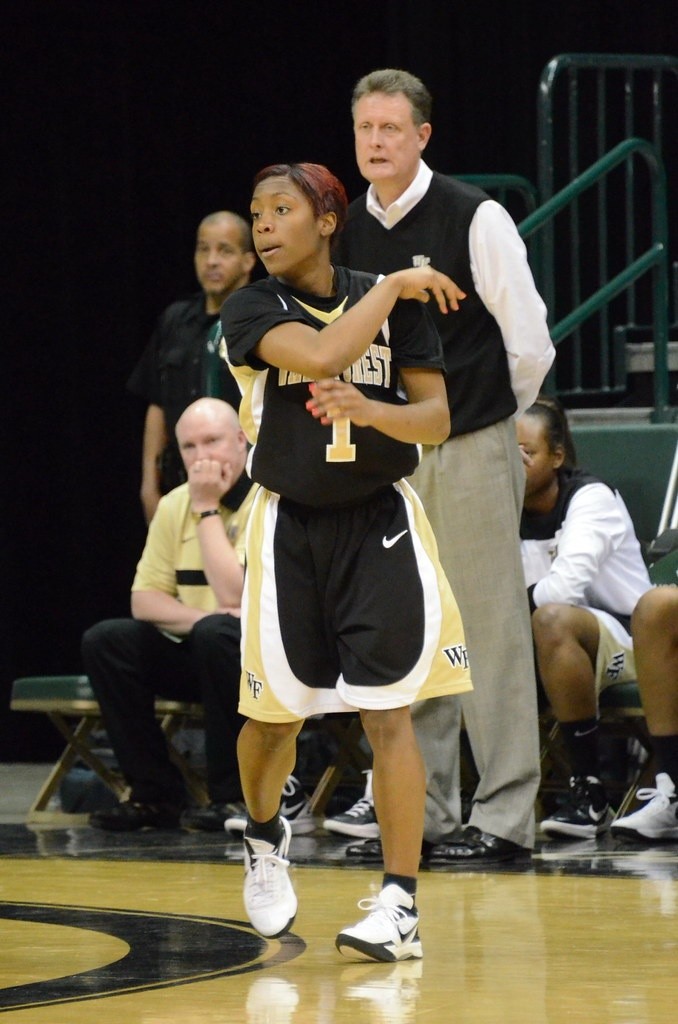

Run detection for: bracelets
[[193, 508, 218, 523]]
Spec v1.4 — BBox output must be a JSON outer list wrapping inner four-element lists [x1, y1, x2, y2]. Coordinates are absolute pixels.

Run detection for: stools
[[10, 675, 213, 825], [533, 679, 658, 827], [296, 711, 373, 819]]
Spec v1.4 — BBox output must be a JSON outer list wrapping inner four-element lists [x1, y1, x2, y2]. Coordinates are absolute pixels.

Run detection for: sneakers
[[222, 774, 317, 838], [238, 814, 299, 940], [611, 772, 678, 845], [538, 774, 617, 838], [333, 882, 424, 962], [323, 769, 382, 843]]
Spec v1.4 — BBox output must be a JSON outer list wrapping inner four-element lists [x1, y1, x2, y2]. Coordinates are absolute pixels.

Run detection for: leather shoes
[[345, 828, 433, 862], [88, 800, 161, 833], [190, 800, 229, 831], [429, 826, 530, 864]]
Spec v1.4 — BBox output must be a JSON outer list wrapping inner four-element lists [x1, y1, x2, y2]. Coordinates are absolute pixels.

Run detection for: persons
[[343, 69, 559, 874], [516, 402, 678, 846], [86, 212, 380, 840], [219, 162, 468, 965]]
